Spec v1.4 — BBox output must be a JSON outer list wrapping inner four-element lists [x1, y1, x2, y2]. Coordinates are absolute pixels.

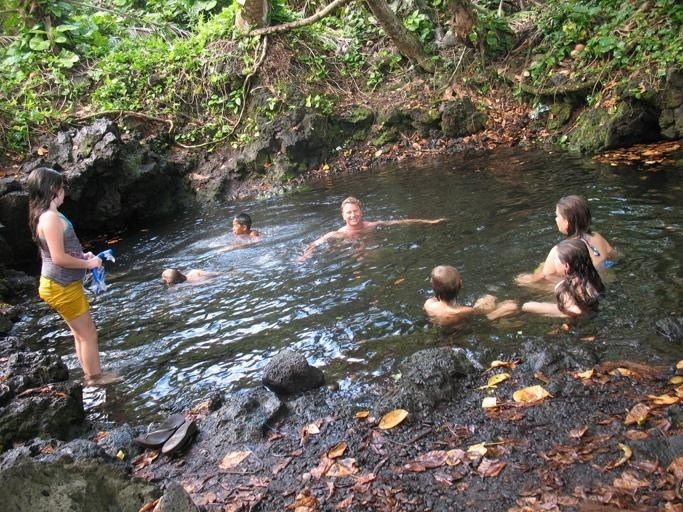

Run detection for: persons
[[514, 194, 621, 293], [297, 195, 447, 266], [161, 268, 221, 287], [26, 167, 123, 387], [521, 240, 606, 319], [426, 266, 517, 333], [221, 213, 259, 253]]
